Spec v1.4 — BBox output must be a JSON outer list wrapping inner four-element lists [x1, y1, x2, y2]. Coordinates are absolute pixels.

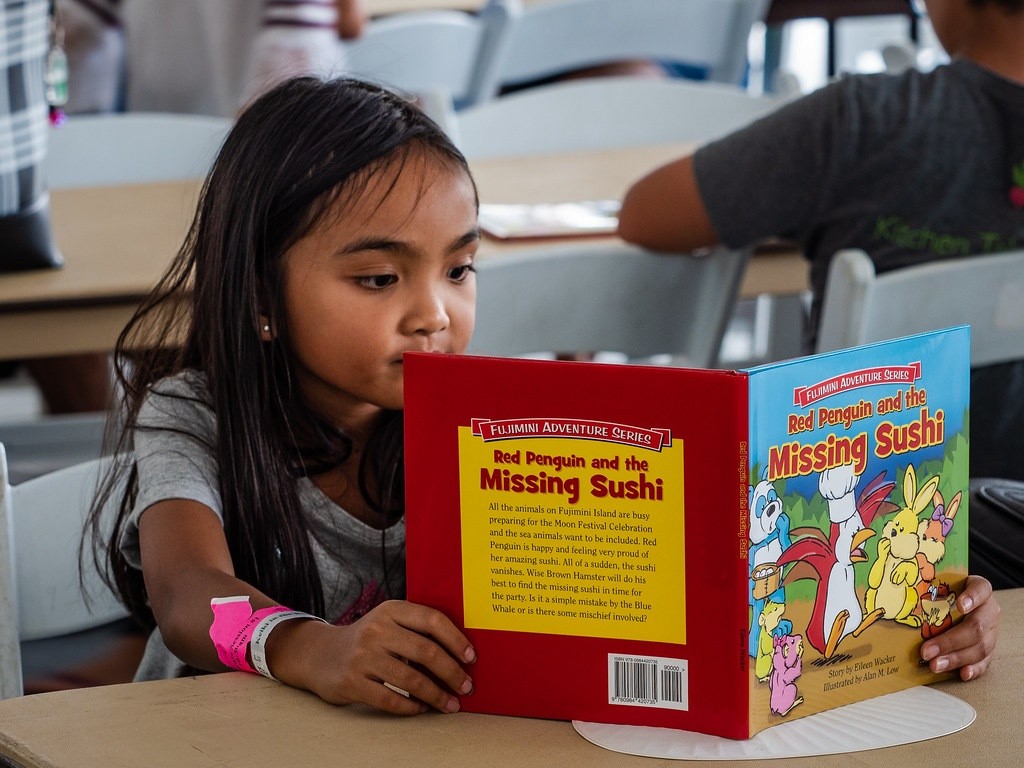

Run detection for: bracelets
[[207, 594, 331, 683]]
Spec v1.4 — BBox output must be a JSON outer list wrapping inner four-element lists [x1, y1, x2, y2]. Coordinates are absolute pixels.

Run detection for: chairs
[[444, 239, 758, 363], [815, 247, 1024, 387], [496, 0, 770, 93], [0, 443, 136, 701], [338, 0, 523, 101]]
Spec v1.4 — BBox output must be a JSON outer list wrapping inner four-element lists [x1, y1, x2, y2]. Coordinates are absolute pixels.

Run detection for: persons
[[240, 0, 365, 107], [74, 67, 1002, 718], [613, 0, 1024, 484]]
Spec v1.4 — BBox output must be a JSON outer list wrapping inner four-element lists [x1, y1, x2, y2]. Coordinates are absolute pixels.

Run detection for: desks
[[0, 144, 1024, 768]]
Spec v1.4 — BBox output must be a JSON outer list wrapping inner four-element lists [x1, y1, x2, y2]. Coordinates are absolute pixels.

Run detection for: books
[[404, 324, 970, 740]]
